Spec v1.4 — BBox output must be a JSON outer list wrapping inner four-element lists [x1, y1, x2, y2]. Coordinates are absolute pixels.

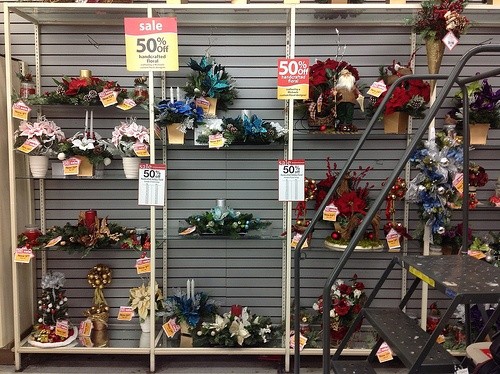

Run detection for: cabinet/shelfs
[[4, 1, 167, 371], [151, 2, 292, 372], [291, 6, 417, 370], [405, 4, 500, 356]]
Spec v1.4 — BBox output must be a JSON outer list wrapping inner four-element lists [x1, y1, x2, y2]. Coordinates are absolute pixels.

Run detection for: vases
[[29, 156, 48, 177], [74, 154, 95, 176], [167, 123, 184, 144], [196, 97, 218, 119], [180, 319, 205, 334], [468, 122, 490, 146], [139, 315, 150, 333], [195, 124, 212, 145], [383, 111, 407, 134], [122, 158, 141, 179], [426, 37, 445, 97]]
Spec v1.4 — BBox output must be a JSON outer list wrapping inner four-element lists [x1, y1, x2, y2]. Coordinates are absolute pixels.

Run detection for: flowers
[[162, 277, 279, 345], [412, 1, 469, 48], [299, 271, 367, 338], [295, 157, 409, 247], [158, 45, 288, 146], [14, 68, 149, 159], [298, 41, 429, 133], [18, 209, 161, 344], [410, 80, 500, 348], [179, 199, 270, 236]]
